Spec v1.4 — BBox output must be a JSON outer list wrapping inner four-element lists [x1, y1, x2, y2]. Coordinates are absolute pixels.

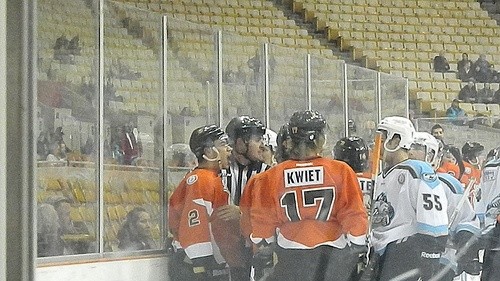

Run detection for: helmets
[[262, 129, 278, 149], [189, 124, 224, 158], [333, 136, 369, 173], [278, 123, 290, 135], [289, 110, 326, 129], [462, 141, 484, 154], [167, 142, 195, 162], [225, 115, 266, 138], [413, 132, 438, 165], [376, 115, 417, 153]]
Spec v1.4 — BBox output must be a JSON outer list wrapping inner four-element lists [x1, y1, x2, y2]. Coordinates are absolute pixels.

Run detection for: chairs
[[38, 0, 500, 254]]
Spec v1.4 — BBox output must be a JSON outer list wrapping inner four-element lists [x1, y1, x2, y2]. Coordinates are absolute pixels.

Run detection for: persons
[[36, 127, 82, 167], [36, 196, 91, 257], [53, 33, 81, 65], [80, 57, 127, 102], [168, 110, 500, 281], [445, 99, 474, 128], [116, 207, 151, 251], [110, 121, 143, 166], [433, 50, 455, 72], [247, 49, 261, 88], [179, 106, 192, 116], [456, 53, 500, 104]]
